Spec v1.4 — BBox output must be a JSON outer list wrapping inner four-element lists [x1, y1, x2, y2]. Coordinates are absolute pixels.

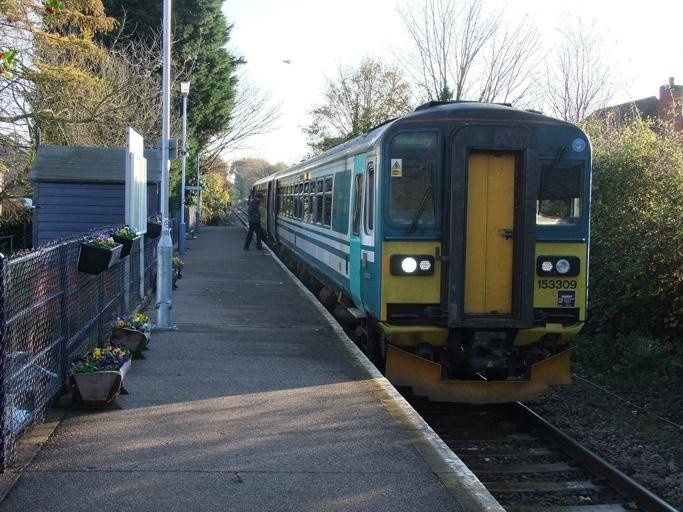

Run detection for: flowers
[[71, 214, 185, 372]]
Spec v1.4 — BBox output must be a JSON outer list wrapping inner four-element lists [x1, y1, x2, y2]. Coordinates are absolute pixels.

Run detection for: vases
[[69, 350, 132, 404]]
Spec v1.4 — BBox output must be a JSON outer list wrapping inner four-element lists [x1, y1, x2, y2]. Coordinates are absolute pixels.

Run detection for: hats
[[254, 193, 264, 199]]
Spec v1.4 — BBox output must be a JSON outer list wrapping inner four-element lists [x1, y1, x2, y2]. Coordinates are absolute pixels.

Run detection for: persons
[[243, 192, 265, 252]]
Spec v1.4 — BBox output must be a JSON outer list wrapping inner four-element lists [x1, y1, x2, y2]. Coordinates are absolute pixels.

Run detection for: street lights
[[177, 77, 192, 256], [196, 150, 202, 236]]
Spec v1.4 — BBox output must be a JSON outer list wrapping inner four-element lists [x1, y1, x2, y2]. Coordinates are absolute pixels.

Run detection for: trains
[[244, 99, 592, 409]]
